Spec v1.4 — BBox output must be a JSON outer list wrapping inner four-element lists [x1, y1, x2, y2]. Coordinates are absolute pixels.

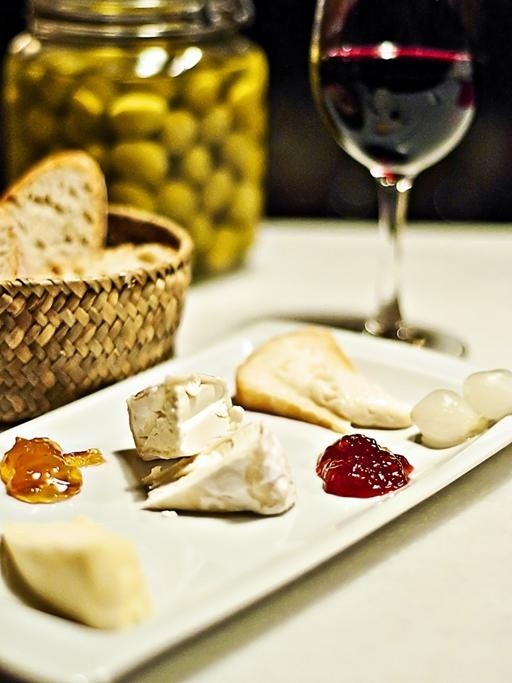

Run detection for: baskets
[[0, 206, 194, 421]]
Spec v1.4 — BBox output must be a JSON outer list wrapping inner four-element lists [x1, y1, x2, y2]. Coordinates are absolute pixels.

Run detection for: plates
[[0, 317, 512, 682]]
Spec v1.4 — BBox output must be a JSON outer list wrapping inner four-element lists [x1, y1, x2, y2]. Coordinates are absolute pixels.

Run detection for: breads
[[143, 420, 296, 516], [127, 372, 243, 461], [3, 518, 144, 631], [1, 150, 175, 279], [236, 328, 411, 432]]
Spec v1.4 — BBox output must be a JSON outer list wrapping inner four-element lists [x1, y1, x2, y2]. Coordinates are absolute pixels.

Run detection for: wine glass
[[307, 0, 482, 362]]
